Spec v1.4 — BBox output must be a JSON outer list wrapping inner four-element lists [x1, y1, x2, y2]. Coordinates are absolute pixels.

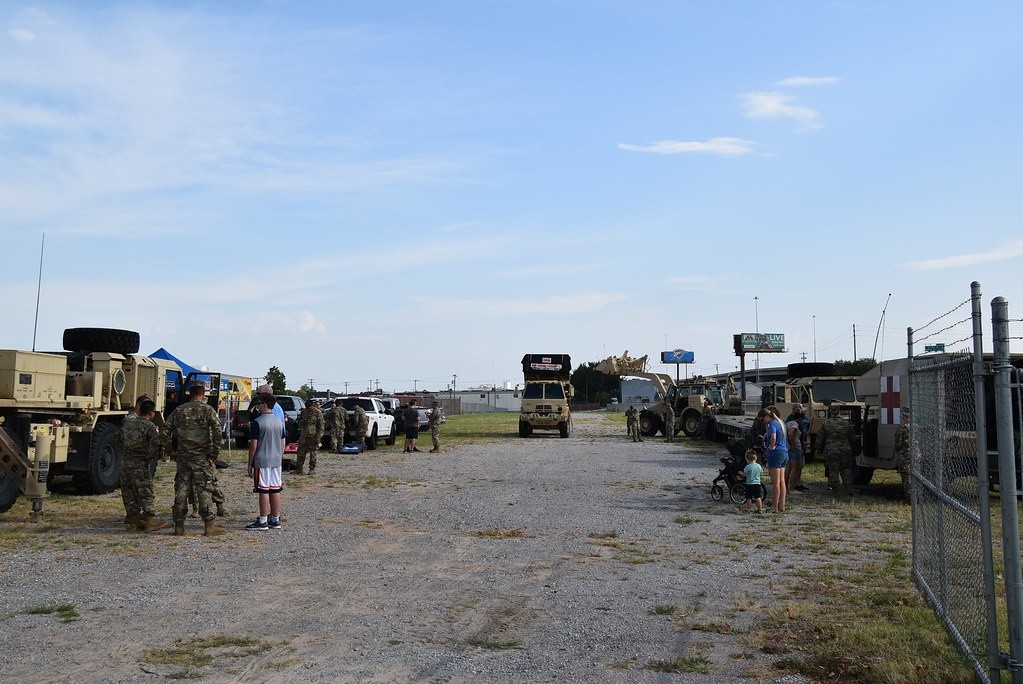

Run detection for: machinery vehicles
[[594, 352, 719, 440], [0, 325, 222, 514]]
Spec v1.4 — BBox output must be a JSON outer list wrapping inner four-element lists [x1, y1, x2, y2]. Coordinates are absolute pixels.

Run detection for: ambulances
[[824, 353, 1023, 500]]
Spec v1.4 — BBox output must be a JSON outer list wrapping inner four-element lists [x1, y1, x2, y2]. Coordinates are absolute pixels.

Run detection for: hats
[[899, 406, 909, 413]]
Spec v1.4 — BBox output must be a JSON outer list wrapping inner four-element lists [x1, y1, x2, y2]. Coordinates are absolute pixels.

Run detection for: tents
[[145, 348, 230, 458]]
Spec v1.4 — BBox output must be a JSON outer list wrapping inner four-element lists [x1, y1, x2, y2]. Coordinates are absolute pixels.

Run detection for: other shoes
[[754, 510, 762, 514], [407, 447, 419, 452], [794, 484, 809, 491], [308, 468, 316, 475], [289, 466, 303, 475], [634, 439, 637, 442], [639, 438, 644, 442]]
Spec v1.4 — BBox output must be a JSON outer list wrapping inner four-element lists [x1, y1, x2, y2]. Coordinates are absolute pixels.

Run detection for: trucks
[[717, 375, 860, 466], [517, 354, 574, 438]]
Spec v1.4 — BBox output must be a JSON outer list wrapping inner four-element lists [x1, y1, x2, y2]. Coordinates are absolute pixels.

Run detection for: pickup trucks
[[233, 395, 309, 448], [320, 397, 397, 448], [382, 398, 446, 431]]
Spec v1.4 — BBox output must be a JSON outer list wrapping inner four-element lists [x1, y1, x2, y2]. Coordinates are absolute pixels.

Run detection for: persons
[[289, 400, 324, 475], [314, 401, 368, 454], [401, 399, 421, 453], [160, 386, 226, 536], [625, 405, 644, 442], [424, 401, 440, 453], [758, 408, 789, 513], [895, 406, 925, 505], [697, 401, 711, 440], [120, 380, 231, 527], [664, 402, 675, 443], [245, 385, 285, 530], [814, 409, 857, 497], [750, 402, 810, 495], [737, 448, 763, 514], [111, 400, 169, 532]]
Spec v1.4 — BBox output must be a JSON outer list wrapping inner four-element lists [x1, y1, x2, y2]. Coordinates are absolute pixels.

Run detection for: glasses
[[258, 402, 264, 405], [257, 392, 266, 396], [761, 415, 765, 422]]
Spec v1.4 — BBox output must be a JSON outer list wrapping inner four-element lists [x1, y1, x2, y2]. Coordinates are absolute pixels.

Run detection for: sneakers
[[256, 514, 282, 529], [243, 520, 268, 531]]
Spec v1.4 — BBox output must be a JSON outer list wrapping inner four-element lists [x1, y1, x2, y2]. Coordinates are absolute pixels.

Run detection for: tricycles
[[710, 456, 767, 504]]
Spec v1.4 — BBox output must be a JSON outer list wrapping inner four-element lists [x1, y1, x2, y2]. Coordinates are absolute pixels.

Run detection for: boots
[[216, 503, 230, 517], [124, 512, 169, 533], [430, 446, 439, 453], [174, 521, 185, 536], [204, 521, 224, 537]]
